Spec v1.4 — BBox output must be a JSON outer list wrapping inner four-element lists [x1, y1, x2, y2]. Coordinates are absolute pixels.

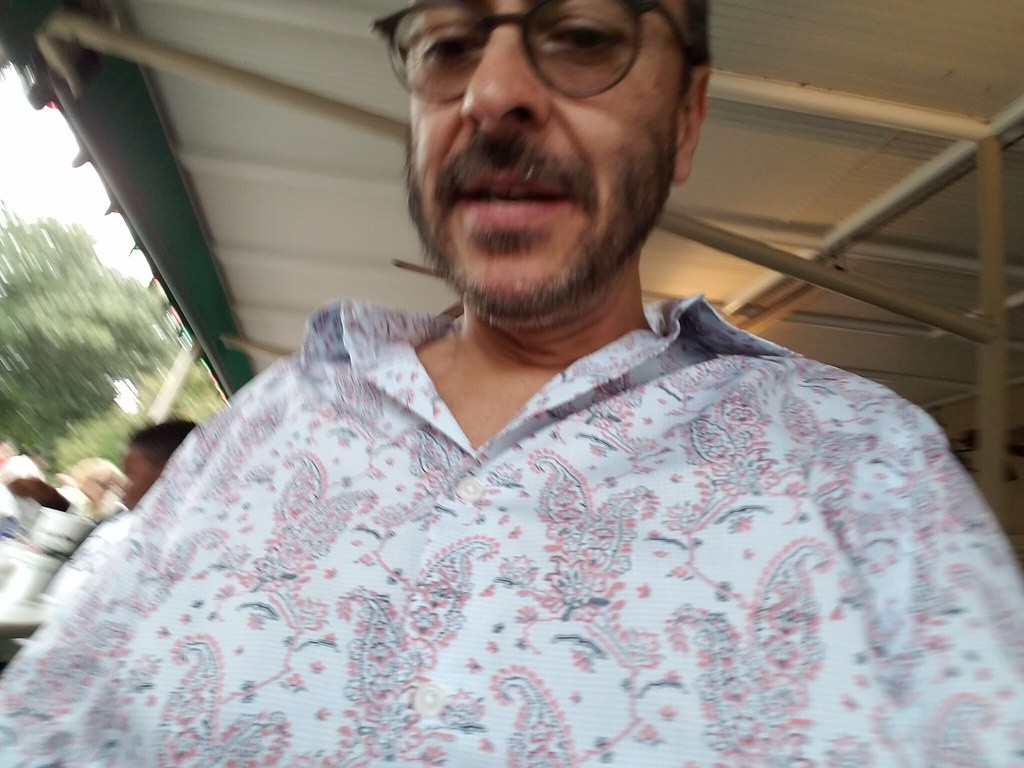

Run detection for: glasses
[[369, 0, 694, 106]]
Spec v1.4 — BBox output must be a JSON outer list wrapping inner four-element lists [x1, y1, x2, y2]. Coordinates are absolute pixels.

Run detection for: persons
[[0, 0, 1024, 768], [0, 418, 198, 616]]
